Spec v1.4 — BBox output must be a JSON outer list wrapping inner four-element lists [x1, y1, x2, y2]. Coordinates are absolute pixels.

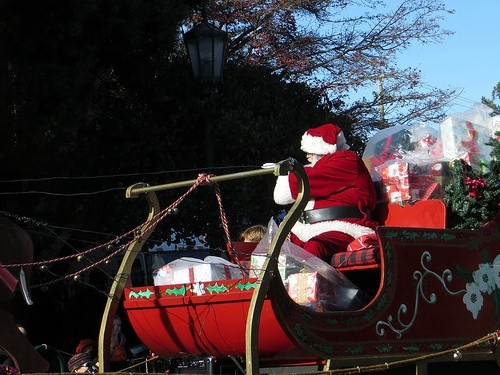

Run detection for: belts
[[300, 206, 375, 224]]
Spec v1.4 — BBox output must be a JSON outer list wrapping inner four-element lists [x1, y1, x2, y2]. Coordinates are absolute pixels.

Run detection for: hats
[[76, 338, 95, 354], [299, 123, 350, 156]]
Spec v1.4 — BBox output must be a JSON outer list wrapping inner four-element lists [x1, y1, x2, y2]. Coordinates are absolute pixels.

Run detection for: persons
[[240, 226, 267, 242], [67, 314, 126, 374], [260, 124, 382, 265]]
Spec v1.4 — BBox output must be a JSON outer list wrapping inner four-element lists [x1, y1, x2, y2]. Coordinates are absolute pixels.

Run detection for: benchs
[[227, 198, 447, 272]]
